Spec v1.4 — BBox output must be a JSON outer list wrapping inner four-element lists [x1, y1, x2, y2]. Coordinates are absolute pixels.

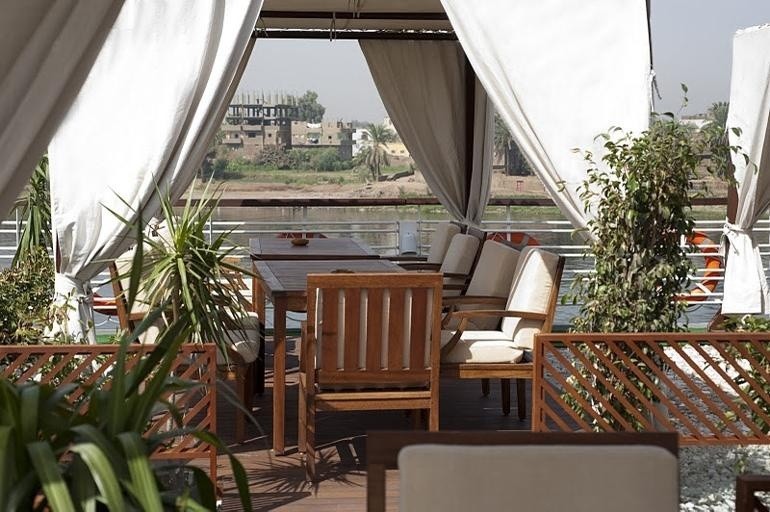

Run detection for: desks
[[245, 236, 411, 454]]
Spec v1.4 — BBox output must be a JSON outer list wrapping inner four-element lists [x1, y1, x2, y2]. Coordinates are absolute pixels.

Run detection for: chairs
[[88, 220, 258, 454], [363, 429, 682, 512], [297, 269, 446, 482], [412, 226, 488, 297], [437, 236, 526, 330], [441, 246, 568, 423], [380, 220, 468, 273]]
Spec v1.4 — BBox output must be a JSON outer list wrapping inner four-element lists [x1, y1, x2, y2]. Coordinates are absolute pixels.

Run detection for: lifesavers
[[274, 231, 327, 238], [660, 234, 721, 306], [88, 288, 128, 316], [485, 231, 540, 247]]
